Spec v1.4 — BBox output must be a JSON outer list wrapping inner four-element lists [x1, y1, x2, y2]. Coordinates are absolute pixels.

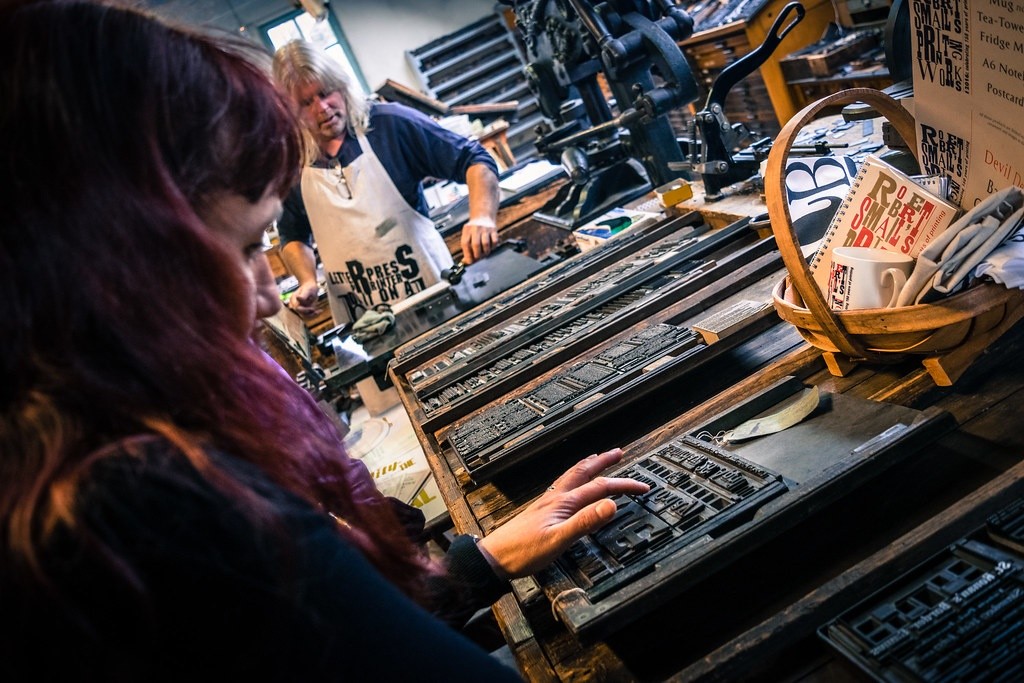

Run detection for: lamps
[[298, 0, 329, 23]]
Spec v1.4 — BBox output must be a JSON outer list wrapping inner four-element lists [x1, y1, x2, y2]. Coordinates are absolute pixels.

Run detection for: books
[[804, 153, 964, 300]]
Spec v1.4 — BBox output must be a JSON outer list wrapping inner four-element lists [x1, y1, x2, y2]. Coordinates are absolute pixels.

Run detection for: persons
[[0, 0, 649, 683], [274, 38, 500, 330]]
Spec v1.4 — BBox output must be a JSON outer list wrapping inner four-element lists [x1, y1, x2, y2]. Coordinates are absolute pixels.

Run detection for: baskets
[[765, 86, 1024, 387]]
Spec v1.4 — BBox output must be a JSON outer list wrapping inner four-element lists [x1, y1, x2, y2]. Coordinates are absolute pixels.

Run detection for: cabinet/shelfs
[[404, 9, 556, 164]]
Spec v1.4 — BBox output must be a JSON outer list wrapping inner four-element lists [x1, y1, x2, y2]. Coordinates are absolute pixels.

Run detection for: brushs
[[441, 258, 466, 285]]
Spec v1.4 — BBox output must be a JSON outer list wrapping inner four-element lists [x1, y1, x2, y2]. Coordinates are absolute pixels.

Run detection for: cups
[[827, 246, 913, 310]]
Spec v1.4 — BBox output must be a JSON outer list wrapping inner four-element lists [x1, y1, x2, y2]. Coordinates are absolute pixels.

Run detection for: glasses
[[328, 158, 353, 200]]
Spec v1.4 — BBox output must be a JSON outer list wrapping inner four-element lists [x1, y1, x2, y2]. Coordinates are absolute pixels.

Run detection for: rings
[[547, 486, 555, 491]]
